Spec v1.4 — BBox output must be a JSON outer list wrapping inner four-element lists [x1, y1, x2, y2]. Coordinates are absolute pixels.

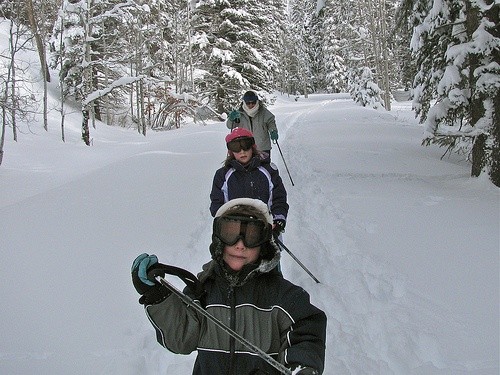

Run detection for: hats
[[243, 91, 258, 102], [215, 198, 273, 228]]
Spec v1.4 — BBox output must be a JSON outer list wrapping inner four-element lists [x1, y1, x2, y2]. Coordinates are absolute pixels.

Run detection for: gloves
[[131, 253, 170, 304], [230, 110, 240, 120], [272, 220, 285, 236], [271, 131, 278, 139]]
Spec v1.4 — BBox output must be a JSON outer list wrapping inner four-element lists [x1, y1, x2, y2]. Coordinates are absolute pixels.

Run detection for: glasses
[[247, 101, 256, 105], [214, 217, 272, 247], [226, 136, 255, 153]]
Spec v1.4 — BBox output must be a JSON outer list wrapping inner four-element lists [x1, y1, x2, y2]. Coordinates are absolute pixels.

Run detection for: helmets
[[225, 127, 257, 148]]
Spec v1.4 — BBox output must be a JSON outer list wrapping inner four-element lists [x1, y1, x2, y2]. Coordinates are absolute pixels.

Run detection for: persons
[[131, 198, 327, 375], [227, 91, 278, 164], [210, 127, 289, 251]]
